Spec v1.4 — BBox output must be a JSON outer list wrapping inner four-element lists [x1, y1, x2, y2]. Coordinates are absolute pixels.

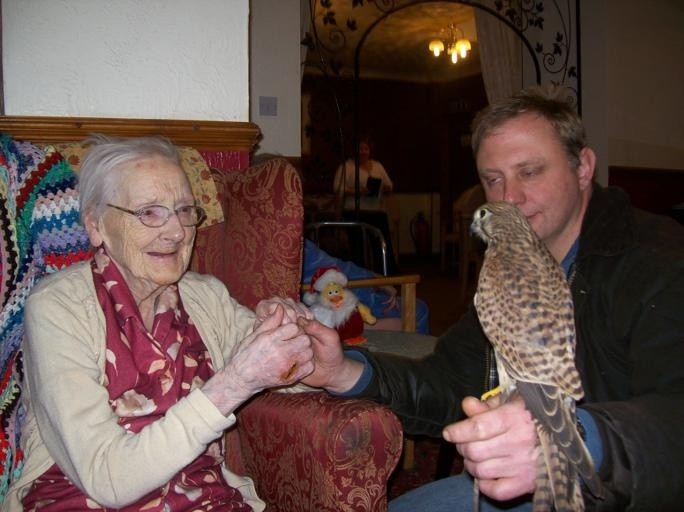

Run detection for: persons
[[0, 140, 314, 512], [333, 136, 403, 278], [297, 96, 682, 510]]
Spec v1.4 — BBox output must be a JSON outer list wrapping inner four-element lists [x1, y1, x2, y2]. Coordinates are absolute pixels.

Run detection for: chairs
[[1, 138, 421, 512]]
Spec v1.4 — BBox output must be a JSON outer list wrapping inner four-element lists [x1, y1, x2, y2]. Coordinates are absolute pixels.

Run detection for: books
[[366, 177, 383, 198]]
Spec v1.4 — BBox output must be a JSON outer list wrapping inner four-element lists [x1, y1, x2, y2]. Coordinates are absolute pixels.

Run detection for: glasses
[[106, 200, 207, 229]]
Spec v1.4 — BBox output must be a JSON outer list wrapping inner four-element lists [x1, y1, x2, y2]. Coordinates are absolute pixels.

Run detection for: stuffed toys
[[304, 266, 376, 345]]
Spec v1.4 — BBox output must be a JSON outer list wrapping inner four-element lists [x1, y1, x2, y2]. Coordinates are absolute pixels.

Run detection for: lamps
[[429, 22, 472, 63]]
